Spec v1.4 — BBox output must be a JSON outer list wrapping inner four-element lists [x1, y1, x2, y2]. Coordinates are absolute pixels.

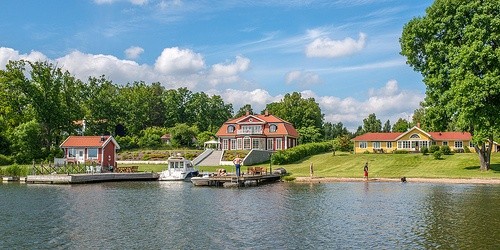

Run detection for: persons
[[216, 169, 227, 176], [364, 163, 369, 180], [233, 155, 243, 176]]
